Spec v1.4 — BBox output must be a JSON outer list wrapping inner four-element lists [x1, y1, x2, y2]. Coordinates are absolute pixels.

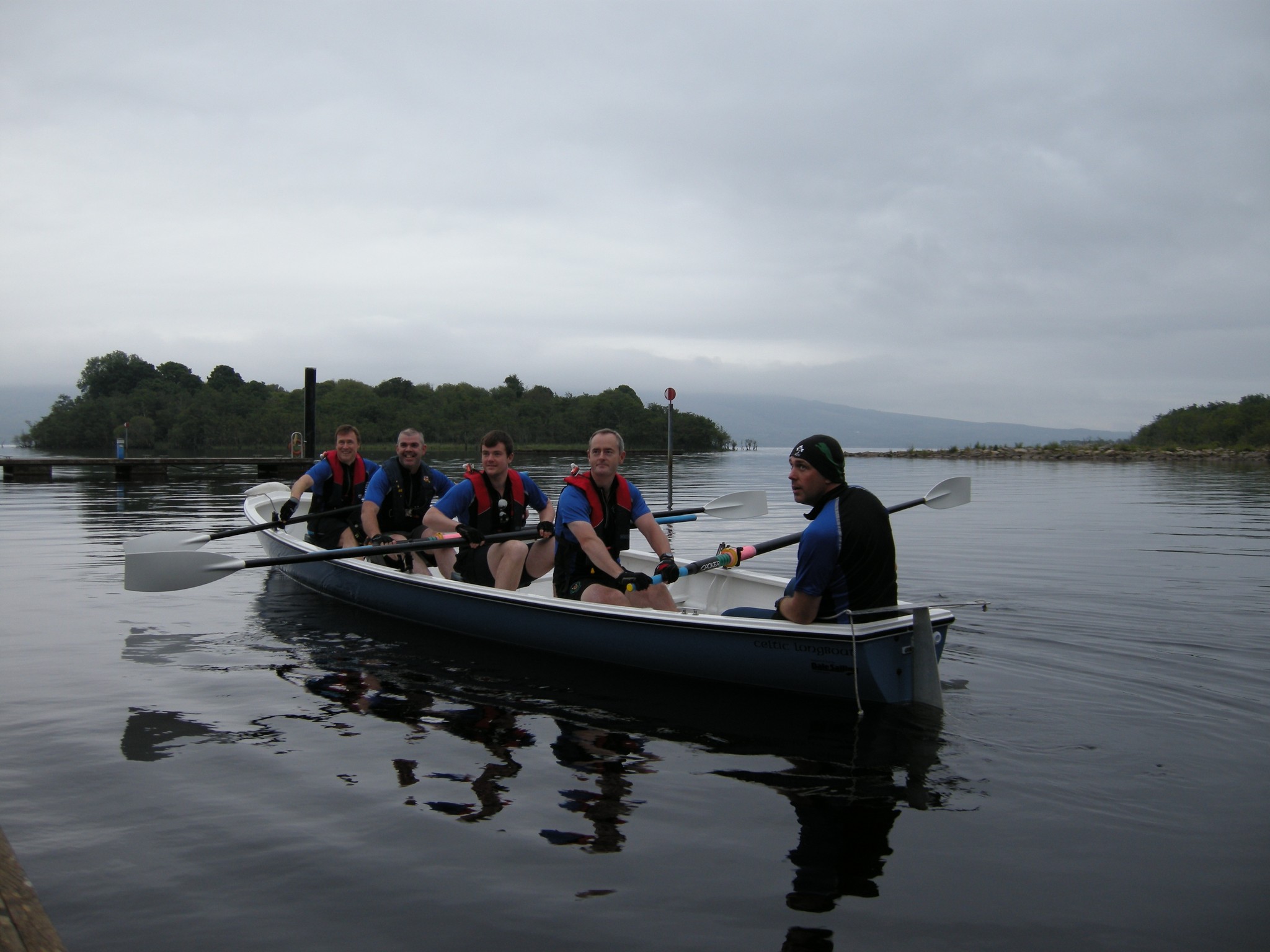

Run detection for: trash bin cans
[[117, 438, 124, 459]]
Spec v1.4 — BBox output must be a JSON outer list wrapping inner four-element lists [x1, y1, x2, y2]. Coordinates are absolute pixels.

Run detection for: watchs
[[659, 552, 674, 561]]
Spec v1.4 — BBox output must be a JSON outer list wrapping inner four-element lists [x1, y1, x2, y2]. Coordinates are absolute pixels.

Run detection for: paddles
[[625, 476, 973, 594], [520, 486, 769, 530], [123, 531, 700, 594], [124, 500, 366, 552]]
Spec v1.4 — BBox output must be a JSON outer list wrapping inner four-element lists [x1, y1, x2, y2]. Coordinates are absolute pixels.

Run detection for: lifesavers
[[288, 439, 305, 455]]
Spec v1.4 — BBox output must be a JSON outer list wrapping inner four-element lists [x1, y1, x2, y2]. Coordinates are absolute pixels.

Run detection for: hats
[[790, 434, 845, 483]]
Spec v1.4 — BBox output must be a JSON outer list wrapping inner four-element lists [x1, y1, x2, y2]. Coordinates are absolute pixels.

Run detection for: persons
[[359, 428, 458, 579], [721, 435, 899, 624], [552, 429, 680, 612], [421, 430, 555, 592], [279, 424, 380, 561]]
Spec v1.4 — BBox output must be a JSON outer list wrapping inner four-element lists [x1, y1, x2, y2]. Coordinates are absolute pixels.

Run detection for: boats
[[241, 480, 955, 714]]
